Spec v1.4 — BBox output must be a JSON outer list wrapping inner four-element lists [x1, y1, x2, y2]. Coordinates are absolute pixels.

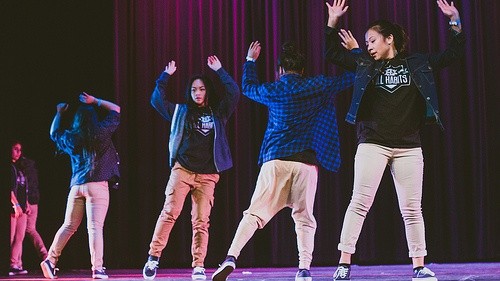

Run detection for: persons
[[143, 55, 241, 280], [18, 155, 48, 270], [322, 0, 464, 281], [8, 143, 31, 275], [40, 91, 121, 279], [212, 29, 365, 281]]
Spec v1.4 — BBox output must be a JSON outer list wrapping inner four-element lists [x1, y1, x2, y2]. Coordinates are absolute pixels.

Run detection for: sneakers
[[333, 264, 351, 281], [9, 267, 28, 275], [41, 259, 59, 279], [295, 269, 311, 281], [192, 267, 206, 280], [93, 267, 107, 278], [412, 267, 438, 281], [143, 256, 160, 280], [212, 257, 235, 281]]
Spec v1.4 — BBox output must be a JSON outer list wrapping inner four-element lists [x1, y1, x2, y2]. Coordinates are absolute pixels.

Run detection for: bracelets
[[98, 98, 101, 106], [246, 56, 256, 63], [449, 19, 461, 26], [15, 204, 20, 206]]
